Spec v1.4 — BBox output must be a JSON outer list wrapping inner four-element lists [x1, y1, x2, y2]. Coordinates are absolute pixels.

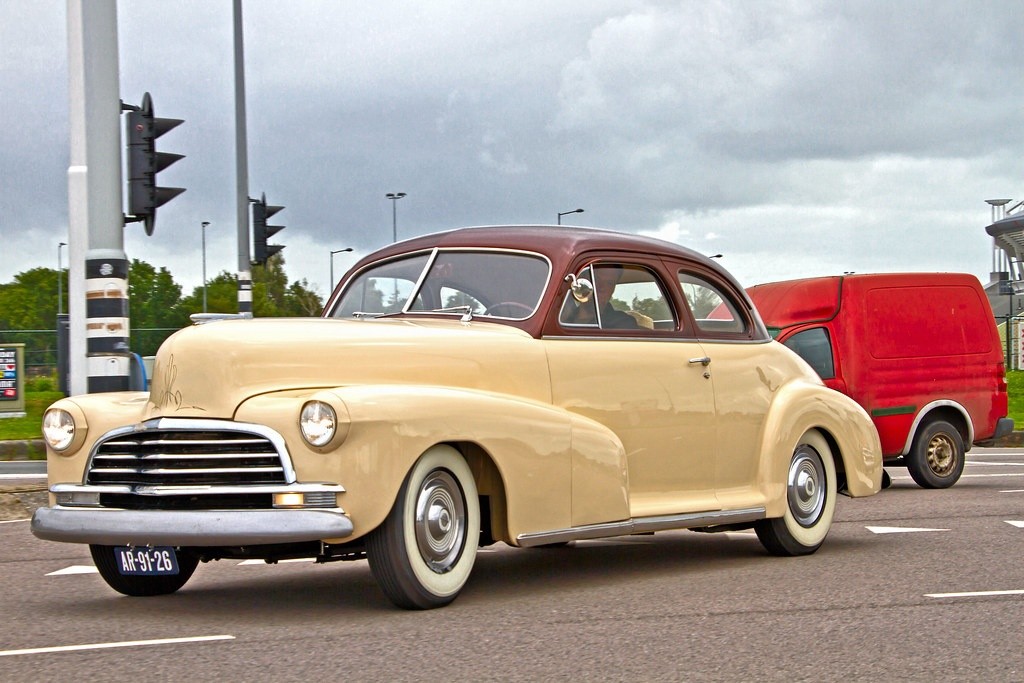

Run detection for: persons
[[563, 262, 639, 331]]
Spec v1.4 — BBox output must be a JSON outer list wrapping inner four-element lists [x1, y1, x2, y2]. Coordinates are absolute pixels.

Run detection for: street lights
[[557, 207, 585, 225], [202, 221, 210, 315], [985, 198, 1013, 278], [329, 247, 353, 300], [385, 191, 407, 307], [57, 242, 67, 313]]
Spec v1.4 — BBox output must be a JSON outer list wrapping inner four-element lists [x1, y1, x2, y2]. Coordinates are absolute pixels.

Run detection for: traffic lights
[[126, 91, 188, 237], [253, 193, 286, 266]]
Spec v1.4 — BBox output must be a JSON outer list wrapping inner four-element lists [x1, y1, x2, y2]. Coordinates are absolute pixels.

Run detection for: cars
[[25, 224, 885, 614]]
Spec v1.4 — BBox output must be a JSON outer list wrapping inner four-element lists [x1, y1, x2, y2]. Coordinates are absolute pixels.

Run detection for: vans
[[702, 271, 1015, 490]]
